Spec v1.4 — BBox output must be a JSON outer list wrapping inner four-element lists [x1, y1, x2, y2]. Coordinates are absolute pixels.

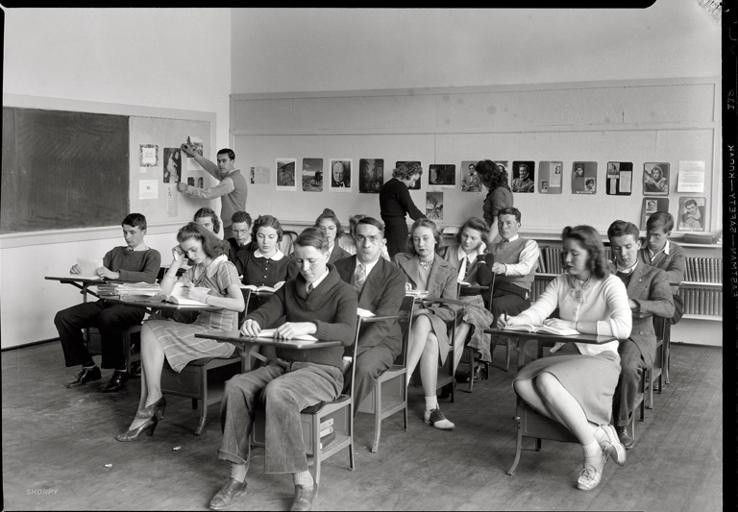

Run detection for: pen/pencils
[[504, 311, 508, 326]]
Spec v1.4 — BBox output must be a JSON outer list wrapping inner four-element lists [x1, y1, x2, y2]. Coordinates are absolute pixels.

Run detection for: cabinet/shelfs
[[532, 271, 723, 326]]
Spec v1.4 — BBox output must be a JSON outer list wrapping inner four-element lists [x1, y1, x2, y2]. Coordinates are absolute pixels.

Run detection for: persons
[[555, 166, 560, 174], [607, 220, 675, 448], [194, 209, 540, 367], [116, 223, 244, 441], [680, 199, 704, 232], [646, 167, 668, 192], [393, 219, 455, 430], [211, 229, 357, 511], [55, 213, 161, 392], [542, 181, 548, 190], [512, 165, 534, 192], [586, 179, 594, 191], [462, 164, 480, 191], [331, 162, 349, 188], [475, 160, 513, 223], [640, 211, 685, 390], [647, 201, 657, 210], [332, 218, 404, 417], [379, 163, 426, 256], [177, 144, 248, 226], [576, 165, 583, 177], [497, 226, 626, 491]]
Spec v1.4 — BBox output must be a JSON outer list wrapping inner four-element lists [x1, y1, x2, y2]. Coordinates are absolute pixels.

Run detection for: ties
[[352, 263, 366, 293], [307, 283, 314, 294]]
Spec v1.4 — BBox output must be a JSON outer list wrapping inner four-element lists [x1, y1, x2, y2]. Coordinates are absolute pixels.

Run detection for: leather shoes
[[423, 408, 456, 430], [614, 421, 636, 450], [467, 366, 488, 382], [64, 364, 102, 389], [208, 477, 249, 511], [575, 450, 606, 491], [595, 422, 628, 466], [289, 476, 320, 511], [103, 366, 129, 392]]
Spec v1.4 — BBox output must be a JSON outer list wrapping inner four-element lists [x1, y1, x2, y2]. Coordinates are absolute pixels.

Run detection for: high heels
[[434, 379, 459, 404], [115, 416, 157, 441], [135, 394, 167, 420]]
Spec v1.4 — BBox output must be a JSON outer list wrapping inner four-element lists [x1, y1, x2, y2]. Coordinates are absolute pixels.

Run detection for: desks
[[194, 330, 344, 350], [255, 291, 276, 300], [120, 299, 223, 311], [413, 297, 447, 305], [362, 314, 401, 324], [484, 326, 616, 345], [461, 284, 490, 294], [44, 275, 125, 354], [631, 312, 654, 321]]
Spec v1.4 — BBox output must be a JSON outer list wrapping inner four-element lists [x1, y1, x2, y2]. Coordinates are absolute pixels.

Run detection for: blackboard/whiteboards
[[0, 93, 217, 250]]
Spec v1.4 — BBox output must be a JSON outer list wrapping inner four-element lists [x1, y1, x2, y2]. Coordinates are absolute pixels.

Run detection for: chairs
[[357, 295, 414, 453], [99, 267, 173, 378], [162, 288, 253, 434], [409, 282, 461, 407], [507, 338, 623, 476], [649, 316, 667, 410], [464, 272, 497, 392], [633, 365, 647, 442], [247, 316, 361, 484], [506, 339, 525, 370]]
